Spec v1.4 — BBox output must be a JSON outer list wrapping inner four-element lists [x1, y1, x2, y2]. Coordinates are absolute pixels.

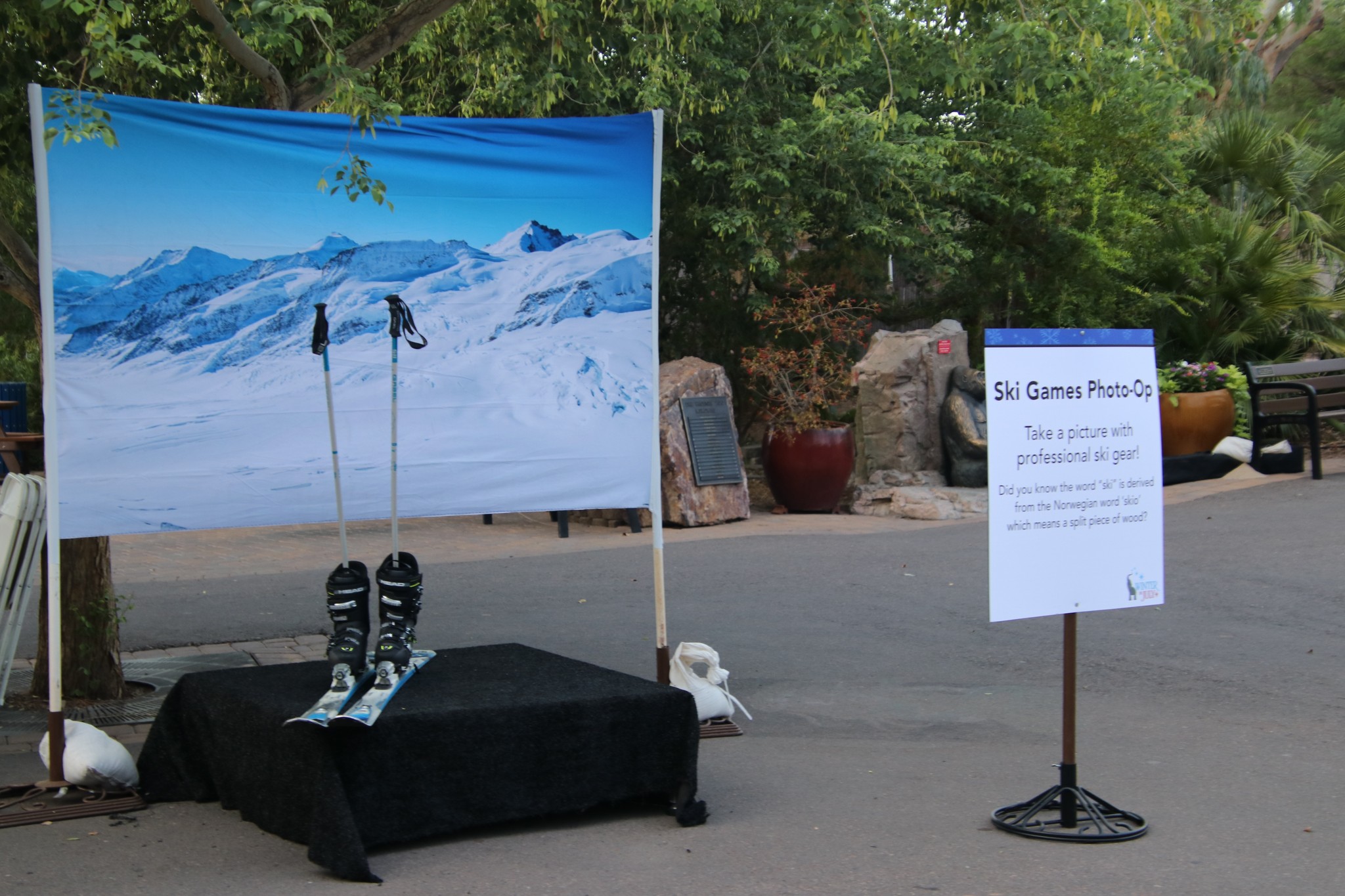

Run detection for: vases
[[1158, 385, 1232, 455], [761, 418, 857, 514]]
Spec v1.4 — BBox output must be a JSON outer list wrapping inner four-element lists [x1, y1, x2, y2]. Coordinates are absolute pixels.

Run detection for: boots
[[321, 561, 372, 678], [374, 552, 424, 674]]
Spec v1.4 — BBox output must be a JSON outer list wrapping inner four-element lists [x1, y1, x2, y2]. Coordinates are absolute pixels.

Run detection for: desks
[[0, 399, 24, 474]]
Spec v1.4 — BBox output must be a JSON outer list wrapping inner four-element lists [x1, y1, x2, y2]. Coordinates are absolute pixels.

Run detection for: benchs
[[1245, 354, 1345, 480], [0, 428, 44, 443]]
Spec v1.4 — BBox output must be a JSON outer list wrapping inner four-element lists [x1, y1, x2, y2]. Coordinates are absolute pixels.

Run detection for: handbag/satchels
[[669, 640, 753, 723]]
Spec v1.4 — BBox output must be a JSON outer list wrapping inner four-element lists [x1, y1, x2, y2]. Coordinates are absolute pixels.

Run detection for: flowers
[[738, 262, 882, 439], [1156, 357, 1252, 440]]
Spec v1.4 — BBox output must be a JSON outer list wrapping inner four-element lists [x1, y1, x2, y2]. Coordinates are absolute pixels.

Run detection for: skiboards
[[282, 650, 438, 730]]
